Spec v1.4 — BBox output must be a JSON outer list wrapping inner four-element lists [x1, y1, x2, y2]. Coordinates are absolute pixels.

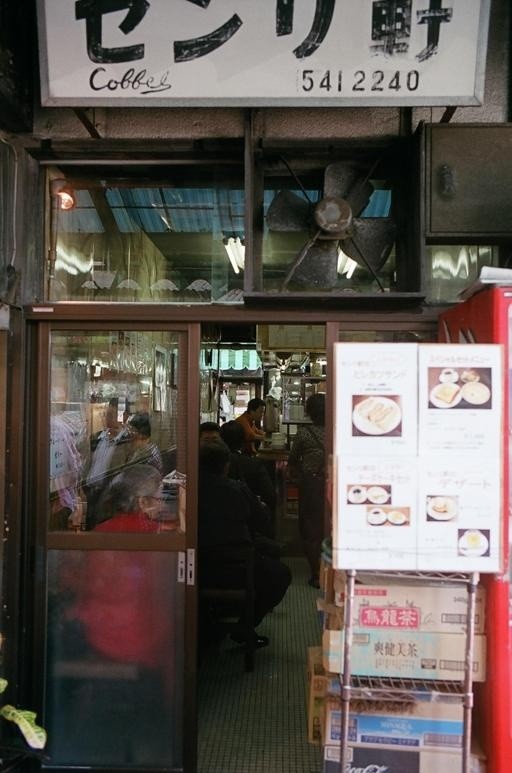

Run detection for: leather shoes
[[230, 630, 269, 648]]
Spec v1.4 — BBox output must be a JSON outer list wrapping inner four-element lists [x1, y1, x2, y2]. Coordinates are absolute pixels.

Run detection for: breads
[[436, 380, 460, 403], [463, 371, 478, 382], [432, 504, 448, 513]]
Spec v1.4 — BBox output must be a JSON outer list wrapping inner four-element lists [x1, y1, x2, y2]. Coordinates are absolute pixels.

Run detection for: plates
[[430, 384, 462, 408], [368, 513, 387, 524], [388, 511, 406, 525], [348, 490, 367, 503], [459, 535, 489, 556], [428, 496, 457, 521], [461, 382, 491, 405], [351, 397, 401, 435], [367, 487, 388, 504], [439, 371, 459, 383]]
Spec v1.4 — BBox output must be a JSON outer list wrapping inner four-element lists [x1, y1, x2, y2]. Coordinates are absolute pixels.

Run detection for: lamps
[[222, 229, 246, 275], [56, 180, 75, 211]]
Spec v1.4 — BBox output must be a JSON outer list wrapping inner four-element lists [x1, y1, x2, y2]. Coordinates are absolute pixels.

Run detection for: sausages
[[358, 397, 397, 430]]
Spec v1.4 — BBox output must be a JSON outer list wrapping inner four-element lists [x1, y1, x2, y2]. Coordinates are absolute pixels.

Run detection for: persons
[[286, 393, 327, 590], [198, 398, 294, 647], [59, 396, 177, 703]]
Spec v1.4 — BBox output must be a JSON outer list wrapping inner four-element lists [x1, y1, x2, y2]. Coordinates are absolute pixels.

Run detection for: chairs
[[197, 539, 256, 669]]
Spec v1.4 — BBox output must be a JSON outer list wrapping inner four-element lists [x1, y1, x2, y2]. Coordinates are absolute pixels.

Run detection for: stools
[[48, 646, 143, 762]]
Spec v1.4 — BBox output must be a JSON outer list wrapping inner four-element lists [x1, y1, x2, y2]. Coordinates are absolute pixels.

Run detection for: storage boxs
[[305, 558, 488, 771]]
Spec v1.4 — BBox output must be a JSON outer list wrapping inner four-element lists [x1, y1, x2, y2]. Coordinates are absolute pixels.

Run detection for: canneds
[[71, 501, 87, 526]]
